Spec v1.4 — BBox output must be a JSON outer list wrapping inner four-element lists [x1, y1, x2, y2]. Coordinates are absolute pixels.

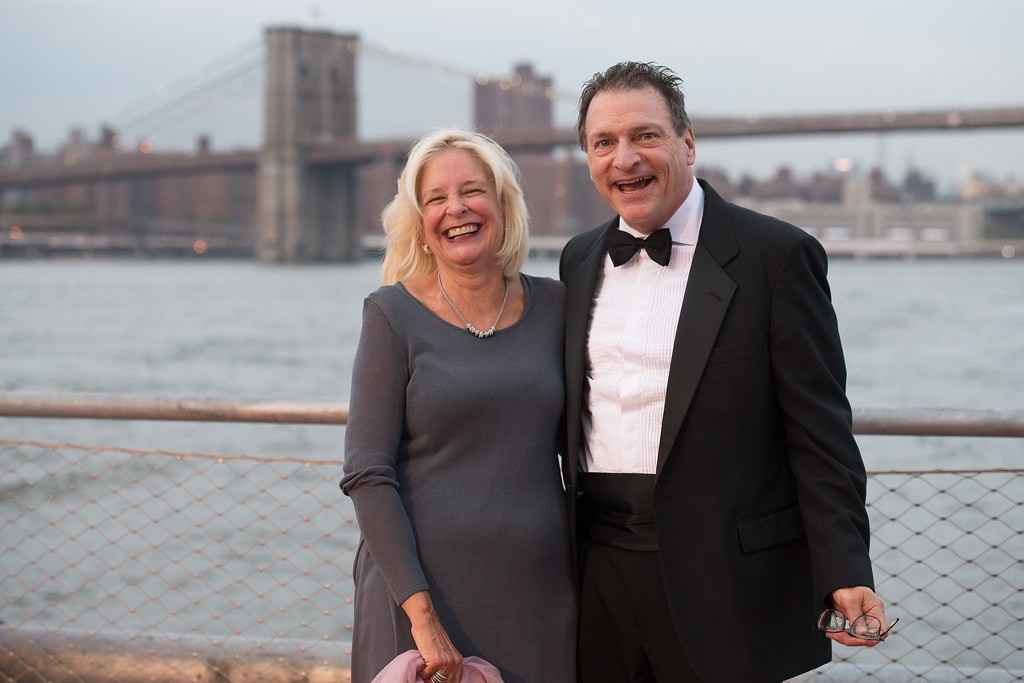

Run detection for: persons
[[340, 129, 576, 683], [560, 61, 888, 683]]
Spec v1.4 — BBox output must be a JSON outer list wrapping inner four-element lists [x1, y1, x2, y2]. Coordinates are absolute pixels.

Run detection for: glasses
[[818, 605, 900, 642]]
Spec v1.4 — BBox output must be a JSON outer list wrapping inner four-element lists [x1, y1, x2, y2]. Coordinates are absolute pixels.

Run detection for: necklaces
[[437, 271, 509, 337]]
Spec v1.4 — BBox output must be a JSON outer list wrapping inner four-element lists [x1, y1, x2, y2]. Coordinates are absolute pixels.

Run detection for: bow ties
[[607, 228, 674, 266]]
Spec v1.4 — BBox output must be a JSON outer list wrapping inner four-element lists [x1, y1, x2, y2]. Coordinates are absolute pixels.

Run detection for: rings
[[431, 672, 447, 683]]
